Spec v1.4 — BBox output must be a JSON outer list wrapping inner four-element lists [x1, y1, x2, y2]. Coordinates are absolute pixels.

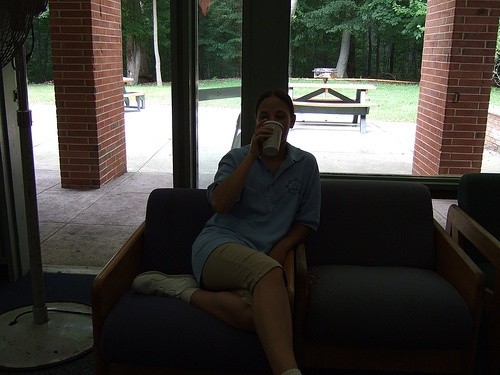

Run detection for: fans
[[0, 0, 94, 368]]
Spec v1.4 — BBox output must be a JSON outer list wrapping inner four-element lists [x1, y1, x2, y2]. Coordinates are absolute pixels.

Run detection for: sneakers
[[131, 271, 200, 299]]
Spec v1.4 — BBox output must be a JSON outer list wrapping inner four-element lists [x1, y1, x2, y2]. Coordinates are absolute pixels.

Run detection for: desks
[[123, 77, 133, 106], [288, 83, 377, 103]]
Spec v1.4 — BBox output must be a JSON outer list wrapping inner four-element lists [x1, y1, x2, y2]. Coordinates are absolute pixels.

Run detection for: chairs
[[123, 90, 150, 112]]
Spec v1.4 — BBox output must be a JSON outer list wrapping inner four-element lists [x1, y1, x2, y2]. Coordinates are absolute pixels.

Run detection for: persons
[[128, 89, 322, 375]]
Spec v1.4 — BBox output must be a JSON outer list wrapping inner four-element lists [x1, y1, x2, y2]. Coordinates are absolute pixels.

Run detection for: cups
[[262, 121, 284, 155]]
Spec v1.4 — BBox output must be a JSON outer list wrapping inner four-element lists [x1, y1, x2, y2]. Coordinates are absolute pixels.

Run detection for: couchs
[[90, 189, 294, 375], [445, 173, 500, 375], [294, 178, 486, 375]]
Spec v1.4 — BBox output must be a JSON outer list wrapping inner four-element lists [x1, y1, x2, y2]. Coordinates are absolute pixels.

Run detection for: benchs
[[293, 96, 370, 127], [289, 101, 380, 133]]
